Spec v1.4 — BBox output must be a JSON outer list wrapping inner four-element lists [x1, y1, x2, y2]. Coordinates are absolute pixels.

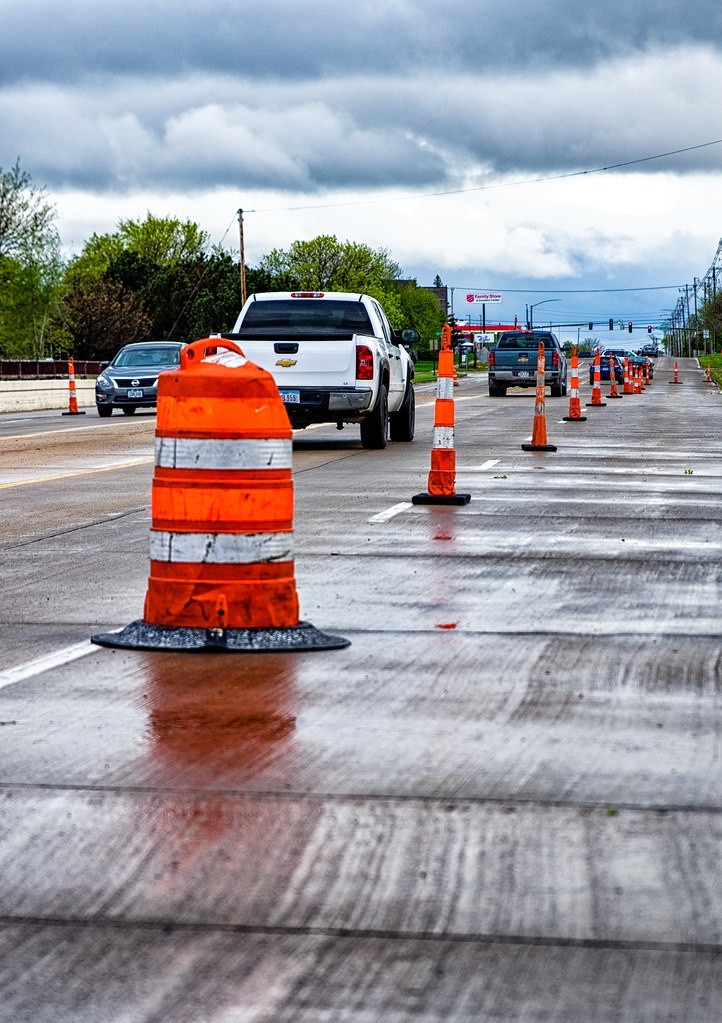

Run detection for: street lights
[[531, 298, 563, 329]]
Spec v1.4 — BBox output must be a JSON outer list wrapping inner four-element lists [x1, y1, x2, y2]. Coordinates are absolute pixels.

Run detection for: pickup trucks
[[487, 329, 568, 397], [208, 290, 422, 450]]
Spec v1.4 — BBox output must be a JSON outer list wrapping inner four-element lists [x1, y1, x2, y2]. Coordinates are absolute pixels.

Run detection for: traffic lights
[[629, 322, 632, 333], [648, 326, 651, 333]]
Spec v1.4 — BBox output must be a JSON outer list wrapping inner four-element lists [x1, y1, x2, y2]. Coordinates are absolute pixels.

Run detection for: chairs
[[540, 338, 552, 347], [507, 339, 520, 347], [133, 354, 143, 362]]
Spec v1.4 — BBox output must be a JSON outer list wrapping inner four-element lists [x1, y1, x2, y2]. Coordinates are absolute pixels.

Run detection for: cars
[[95, 341, 188, 417], [623, 358, 655, 380], [601, 348, 655, 366], [640, 345, 659, 358], [589, 356, 624, 385]]
